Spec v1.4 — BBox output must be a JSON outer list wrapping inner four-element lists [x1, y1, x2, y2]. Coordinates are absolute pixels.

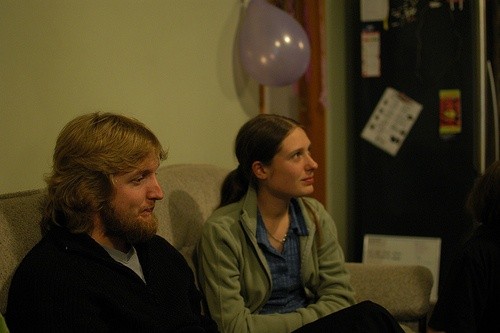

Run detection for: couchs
[[0, 164, 433, 333]]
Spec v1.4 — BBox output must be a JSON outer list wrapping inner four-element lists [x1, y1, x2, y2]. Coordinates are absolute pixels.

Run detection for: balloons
[[238, 1, 309, 88]]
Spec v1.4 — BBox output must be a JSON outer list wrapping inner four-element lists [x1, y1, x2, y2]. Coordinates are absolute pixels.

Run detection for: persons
[[5, 109, 407, 333], [426, 160, 500, 333], [195, 111, 356, 333]]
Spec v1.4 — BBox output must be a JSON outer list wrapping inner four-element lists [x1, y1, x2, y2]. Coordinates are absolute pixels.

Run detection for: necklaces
[[266, 229, 287, 245]]
